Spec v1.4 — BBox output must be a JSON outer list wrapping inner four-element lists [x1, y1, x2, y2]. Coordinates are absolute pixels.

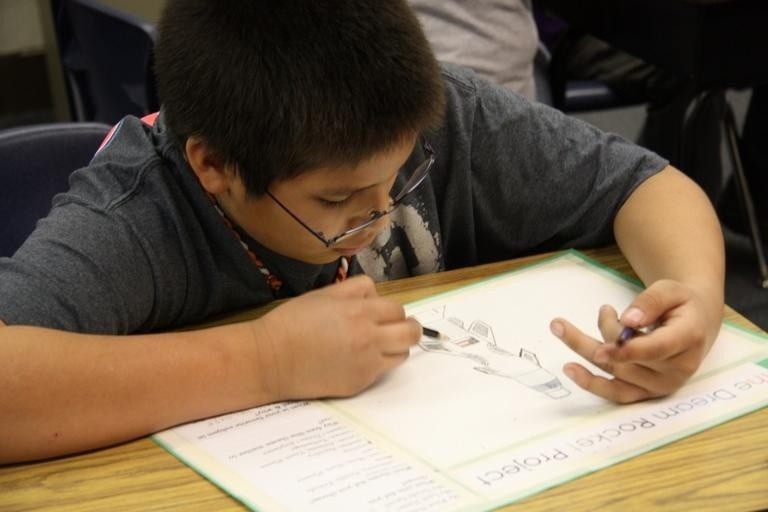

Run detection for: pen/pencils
[[422, 326, 450, 342], [616, 326, 634, 346]]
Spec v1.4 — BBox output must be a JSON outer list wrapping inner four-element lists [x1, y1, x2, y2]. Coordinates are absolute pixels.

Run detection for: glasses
[[262, 133, 435, 247]]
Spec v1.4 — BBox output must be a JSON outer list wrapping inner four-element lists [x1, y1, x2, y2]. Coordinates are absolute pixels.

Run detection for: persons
[[409, 0, 768, 242], [1, 1, 726, 467]]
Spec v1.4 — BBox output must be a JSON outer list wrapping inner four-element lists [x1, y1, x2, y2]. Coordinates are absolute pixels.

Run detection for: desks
[[1, 245, 768, 510]]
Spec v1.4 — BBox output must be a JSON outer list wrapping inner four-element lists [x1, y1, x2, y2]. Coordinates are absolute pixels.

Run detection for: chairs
[[0, 122, 113, 256], [46, 1, 160, 129], [533, 36, 768, 299]]
